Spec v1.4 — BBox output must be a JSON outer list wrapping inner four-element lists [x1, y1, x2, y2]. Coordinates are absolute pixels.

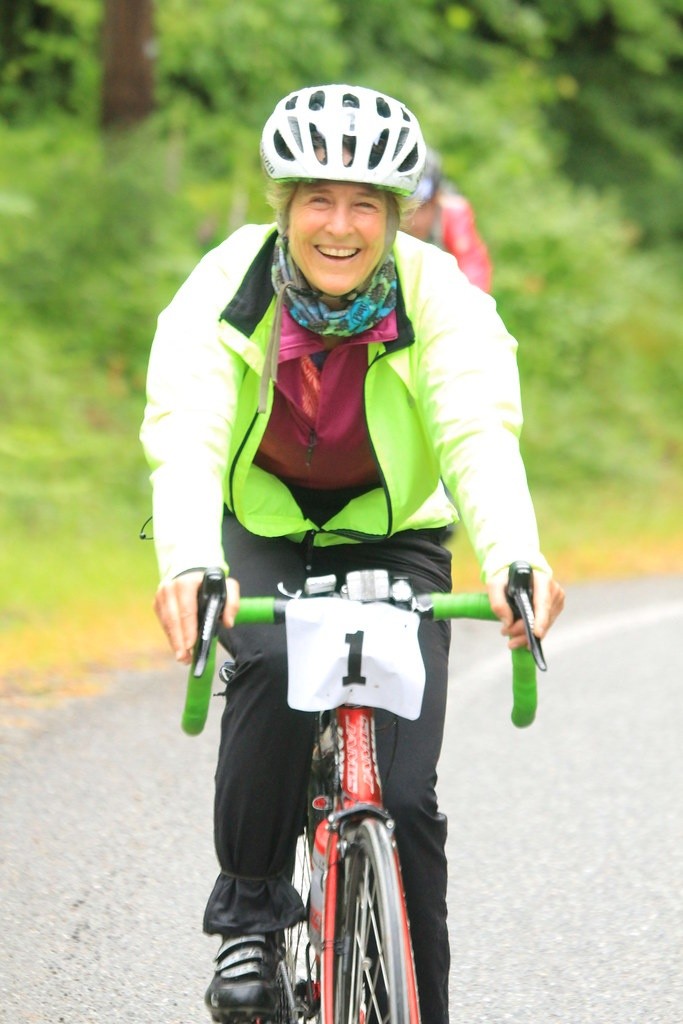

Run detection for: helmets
[[258, 84, 426, 195], [408, 150, 443, 209]]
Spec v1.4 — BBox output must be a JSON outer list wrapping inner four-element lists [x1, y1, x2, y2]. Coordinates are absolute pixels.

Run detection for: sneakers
[[206, 930, 279, 1014]]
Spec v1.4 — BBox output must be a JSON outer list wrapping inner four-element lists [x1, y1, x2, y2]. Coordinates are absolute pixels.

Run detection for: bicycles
[[180, 554, 548, 1024]]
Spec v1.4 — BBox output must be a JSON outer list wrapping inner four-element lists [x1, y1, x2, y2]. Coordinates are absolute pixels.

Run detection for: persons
[[138, 83, 564, 1022], [398, 148, 493, 294]]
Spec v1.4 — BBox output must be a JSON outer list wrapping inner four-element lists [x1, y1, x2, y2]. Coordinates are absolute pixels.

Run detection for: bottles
[[308, 858, 324, 948]]
